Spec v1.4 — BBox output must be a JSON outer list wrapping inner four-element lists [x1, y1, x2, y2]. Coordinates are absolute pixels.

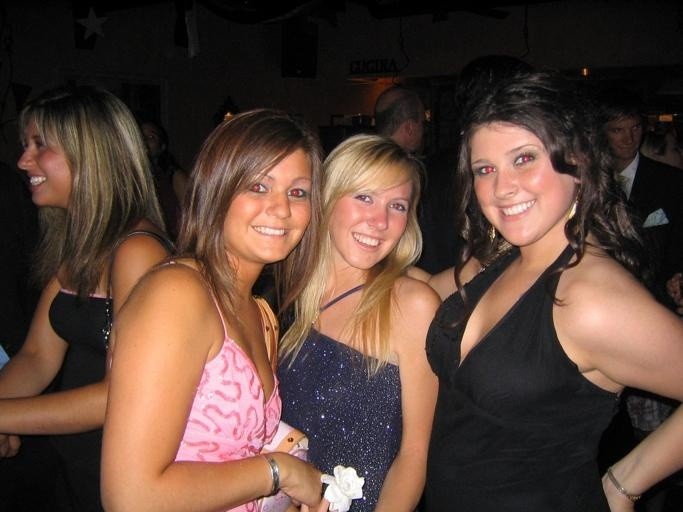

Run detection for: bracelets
[[261, 453, 279, 495], [607, 469, 641, 504]]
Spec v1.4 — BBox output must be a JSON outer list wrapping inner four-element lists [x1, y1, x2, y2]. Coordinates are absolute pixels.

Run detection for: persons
[[667, 273, 683, 316], [599, 103, 683, 439], [375, 85, 454, 275], [644, 124, 683, 168], [0, 343, 22, 459], [401, 74, 683, 512], [267, 131, 442, 512], [138, 110, 230, 233], [0, 85, 176, 512], [99, 108, 334, 512]]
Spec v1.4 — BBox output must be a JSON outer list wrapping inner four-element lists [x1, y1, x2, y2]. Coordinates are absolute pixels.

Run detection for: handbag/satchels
[[257, 419, 310, 510]]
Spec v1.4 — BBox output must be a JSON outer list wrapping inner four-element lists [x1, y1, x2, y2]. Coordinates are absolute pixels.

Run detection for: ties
[[616, 174, 630, 195]]
